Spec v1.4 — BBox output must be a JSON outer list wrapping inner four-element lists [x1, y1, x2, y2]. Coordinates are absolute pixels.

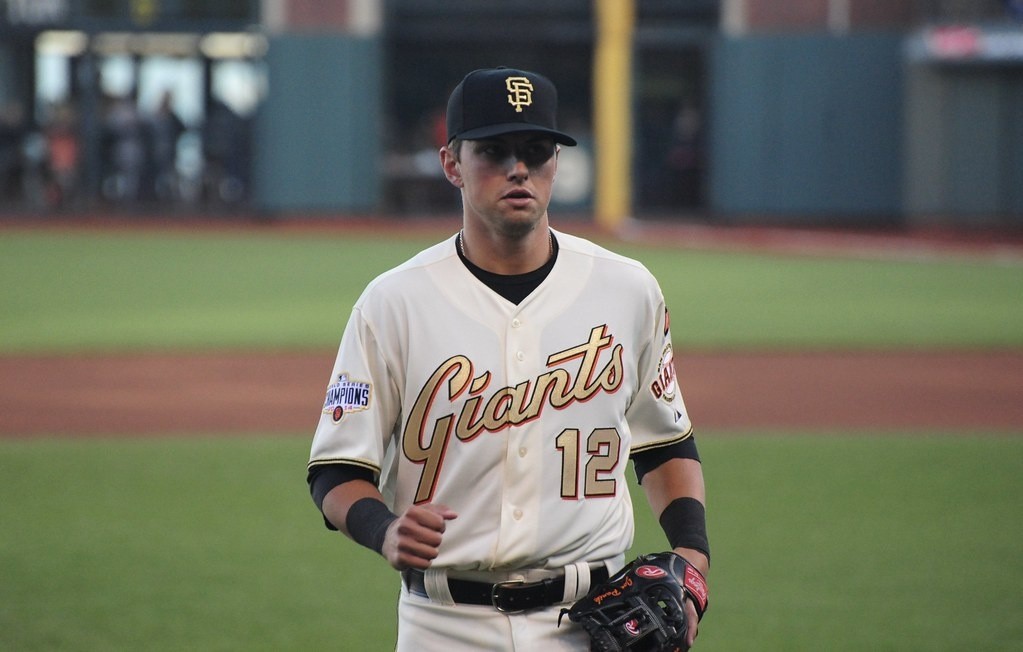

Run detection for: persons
[[307, 66, 711, 652], [1, 92, 185, 210]]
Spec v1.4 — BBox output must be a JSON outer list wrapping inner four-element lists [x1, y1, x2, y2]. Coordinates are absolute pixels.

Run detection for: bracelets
[[346, 497, 398, 555], [659, 497, 710, 569]]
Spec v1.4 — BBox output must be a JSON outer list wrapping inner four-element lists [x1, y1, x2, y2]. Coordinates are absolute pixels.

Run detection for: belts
[[406, 566, 609, 615]]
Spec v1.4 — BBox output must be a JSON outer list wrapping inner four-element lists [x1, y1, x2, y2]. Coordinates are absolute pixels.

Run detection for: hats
[[446, 65, 578, 149]]
